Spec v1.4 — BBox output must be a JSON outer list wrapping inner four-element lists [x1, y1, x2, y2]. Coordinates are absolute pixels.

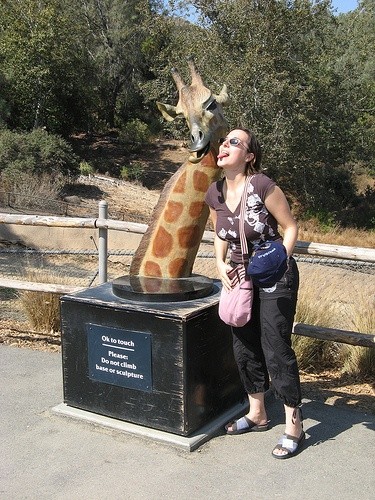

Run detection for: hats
[[247, 240, 289, 289]]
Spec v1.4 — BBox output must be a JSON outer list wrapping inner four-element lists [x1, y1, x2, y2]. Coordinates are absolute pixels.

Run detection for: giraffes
[[128, 55, 233, 280]]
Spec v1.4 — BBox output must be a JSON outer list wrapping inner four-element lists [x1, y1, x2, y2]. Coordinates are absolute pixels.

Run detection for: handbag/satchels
[[217, 265, 253, 328]]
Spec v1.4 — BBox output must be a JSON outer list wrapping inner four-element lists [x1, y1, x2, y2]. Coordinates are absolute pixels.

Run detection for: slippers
[[271, 430, 306, 459], [224, 415, 271, 435]]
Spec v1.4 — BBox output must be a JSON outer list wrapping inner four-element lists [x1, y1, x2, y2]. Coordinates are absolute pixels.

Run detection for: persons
[[205, 128, 305, 459]]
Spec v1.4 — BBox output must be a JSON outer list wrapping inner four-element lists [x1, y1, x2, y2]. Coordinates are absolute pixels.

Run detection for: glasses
[[218, 137, 251, 153]]
